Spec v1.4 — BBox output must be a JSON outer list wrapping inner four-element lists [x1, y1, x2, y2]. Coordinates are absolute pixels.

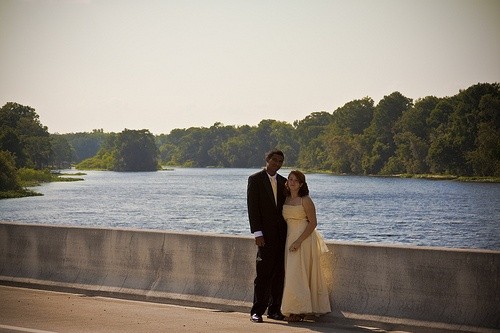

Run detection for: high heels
[[284, 313, 304, 322]]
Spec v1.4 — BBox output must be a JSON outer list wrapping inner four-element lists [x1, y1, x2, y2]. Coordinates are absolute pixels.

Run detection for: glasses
[[269, 157, 283, 164]]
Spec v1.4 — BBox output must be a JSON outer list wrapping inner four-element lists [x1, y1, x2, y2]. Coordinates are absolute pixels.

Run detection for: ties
[[271, 176, 277, 206]]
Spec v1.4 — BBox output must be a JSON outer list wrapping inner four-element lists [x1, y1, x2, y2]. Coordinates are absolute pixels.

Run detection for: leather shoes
[[267, 313, 284, 320], [250, 313, 263, 323]]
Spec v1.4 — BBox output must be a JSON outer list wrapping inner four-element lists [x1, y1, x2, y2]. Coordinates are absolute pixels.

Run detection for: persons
[[247, 150, 288, 323], [282, 169, 331, 323]]
